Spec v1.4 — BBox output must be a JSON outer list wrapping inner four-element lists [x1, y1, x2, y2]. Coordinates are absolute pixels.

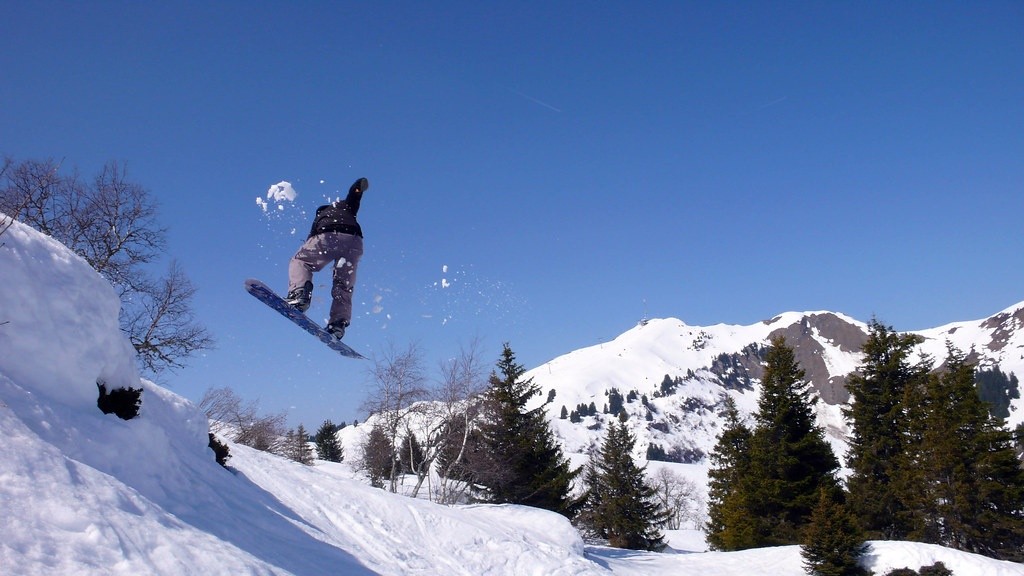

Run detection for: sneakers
[[283, 280, 313, 312], [324, 318, 348, 340]]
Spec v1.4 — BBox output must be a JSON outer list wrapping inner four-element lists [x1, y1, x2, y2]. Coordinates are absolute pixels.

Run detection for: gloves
[[361, 178, 368, 191]]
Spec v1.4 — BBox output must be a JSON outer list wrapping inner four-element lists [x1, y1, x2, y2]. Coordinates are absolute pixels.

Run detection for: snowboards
[[240, 274, 373, 364]]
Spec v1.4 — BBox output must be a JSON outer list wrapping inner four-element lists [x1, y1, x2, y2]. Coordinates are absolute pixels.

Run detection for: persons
[[281, 177, 368, 340]]
